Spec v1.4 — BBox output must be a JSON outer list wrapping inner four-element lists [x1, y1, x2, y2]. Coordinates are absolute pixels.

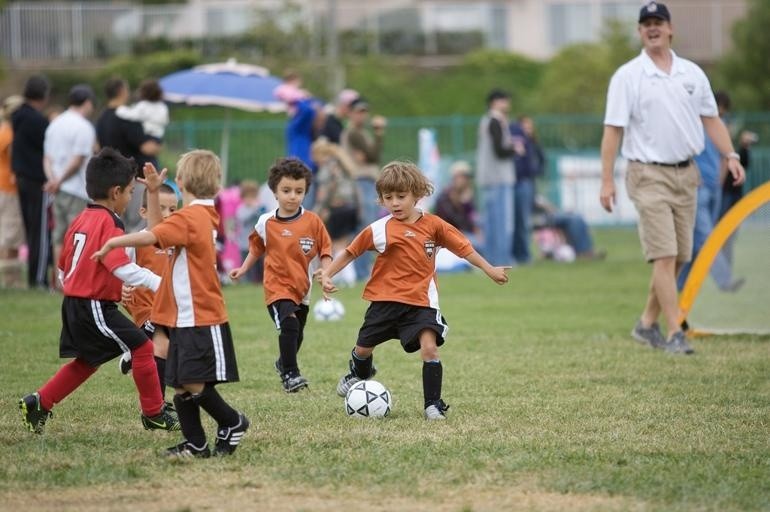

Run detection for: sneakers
[[213, 412, 249, 456], [19, 392, 48, 435], [336, 360, 377, 397], [280, 371, 309, 393], [423, 399, 450, 420], [119, 350, 132, 375], [167, 440, 210, 460], [141, 407, 182, 431], [630, 320, 663, 349], [662, 330, 695, 354]]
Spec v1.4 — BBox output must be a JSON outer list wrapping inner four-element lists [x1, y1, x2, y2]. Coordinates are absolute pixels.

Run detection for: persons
[[18, 145, 188, 434], [310, 135, 361, 247], [531, 193, 606, 259], [230, 156, 334, 394], [600, 2, 747, 356], [284, 99, 319, 212], [677, 89, 745, 295], [313, 91, 357, 147], [109, 80, 168, 174], [274, 69, 317, 112], [235, 178, 270, 285], [120, 162, 182, 417], [0, 94, 27, 288], [719, 121, 757, 267], [343, 99, 387, 285], [11, 76, 53, 286], [323, 158, 512, 422], [42, 85, 98, 284], [95, 73, 161, 229], [90, 149, 248, 465], [476, 89, 525, 273], [435, 162, 483, 233], [510, 113, 544, 265]]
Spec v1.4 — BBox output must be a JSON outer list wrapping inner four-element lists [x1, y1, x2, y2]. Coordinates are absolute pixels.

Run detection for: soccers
[[345, 380, 392, 421], [314, 298, 345, 322], [553, 245, 576, 264]]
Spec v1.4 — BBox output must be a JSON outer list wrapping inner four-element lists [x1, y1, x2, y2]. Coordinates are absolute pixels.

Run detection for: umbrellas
[[157, 58, 296, 186]]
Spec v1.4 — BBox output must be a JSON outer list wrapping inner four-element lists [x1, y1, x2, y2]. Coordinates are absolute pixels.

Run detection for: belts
[[652, 159, 690, 168]]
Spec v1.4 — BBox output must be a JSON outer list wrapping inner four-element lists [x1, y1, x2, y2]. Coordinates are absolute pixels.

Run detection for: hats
[[638, 2, 669, 24]]
